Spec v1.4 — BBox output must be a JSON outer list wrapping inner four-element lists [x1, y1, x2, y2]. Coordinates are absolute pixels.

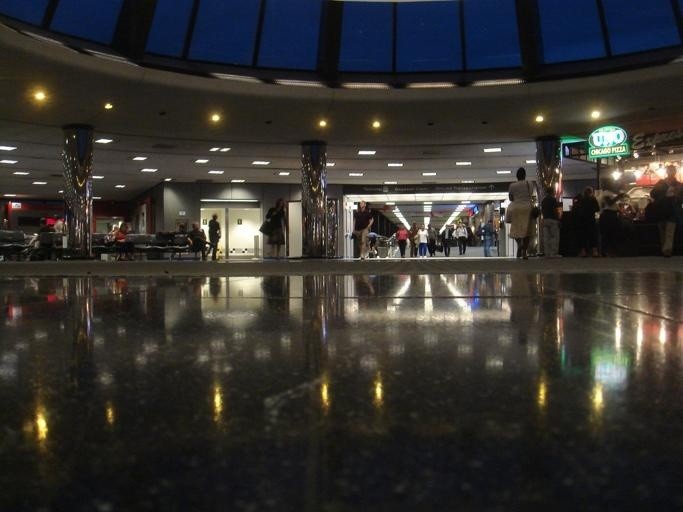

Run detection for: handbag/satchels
[[532, 208, 538, 217], [259, 221, 274, 235]]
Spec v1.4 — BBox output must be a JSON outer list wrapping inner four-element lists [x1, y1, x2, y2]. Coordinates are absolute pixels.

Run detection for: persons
[[351, 200, 498, 260], [265, 198, 288, 258], [104, 223, 134, 257], [24, 213, 66, 255], [169, 213, 222, 259], [507, 166, 683, 259]]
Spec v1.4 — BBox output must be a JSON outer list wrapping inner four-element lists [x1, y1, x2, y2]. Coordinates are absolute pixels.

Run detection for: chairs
[[1, 230, 209, 261]]
